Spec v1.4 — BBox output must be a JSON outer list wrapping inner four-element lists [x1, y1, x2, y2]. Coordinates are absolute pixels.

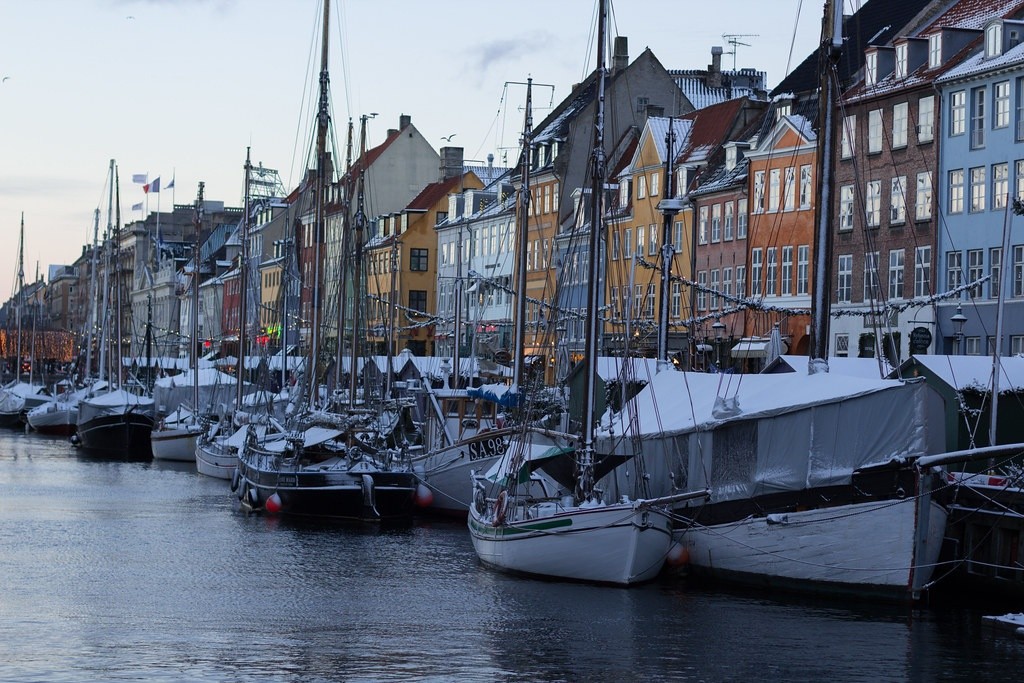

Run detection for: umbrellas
[[556, 340, 568, 379], [765, 322, 781, 366], [399, 348, 414, 357]]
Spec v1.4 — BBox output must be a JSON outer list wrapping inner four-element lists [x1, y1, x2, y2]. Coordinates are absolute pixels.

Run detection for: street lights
[[555, 325, 567, 386], [711, 317, 727, 373], [949, 301, 969, 355]]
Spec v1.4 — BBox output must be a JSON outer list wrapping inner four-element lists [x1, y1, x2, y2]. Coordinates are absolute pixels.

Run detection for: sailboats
[[1, 0, 1024, 635]]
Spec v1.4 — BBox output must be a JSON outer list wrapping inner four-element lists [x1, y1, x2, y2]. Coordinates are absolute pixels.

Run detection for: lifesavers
[[349, 446, 363, 460], [160, 418, 165, 428], [492, 490, 509, 526], [947, 471, 1007, 486], [247, 489, 258, 509], [477, 428, 490, 434]]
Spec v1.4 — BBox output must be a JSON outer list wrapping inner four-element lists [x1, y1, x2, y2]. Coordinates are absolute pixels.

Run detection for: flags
[[143, 177, 160, 193], [132, 202, 142, 210], [133, 174, 147, 184], [164, 179, 174, 189]]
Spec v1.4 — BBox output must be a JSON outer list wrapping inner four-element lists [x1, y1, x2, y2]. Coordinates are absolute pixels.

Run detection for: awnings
[[731, 336, 790, 357], [278, 343, 296, 356], [203, 350, 219, 360]]
